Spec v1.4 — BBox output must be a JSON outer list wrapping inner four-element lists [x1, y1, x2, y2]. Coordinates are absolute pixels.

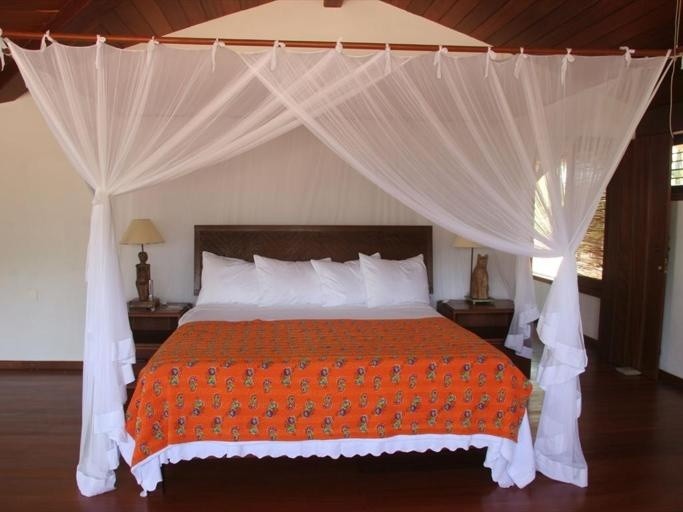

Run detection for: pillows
[[196, 250, 430, 310]]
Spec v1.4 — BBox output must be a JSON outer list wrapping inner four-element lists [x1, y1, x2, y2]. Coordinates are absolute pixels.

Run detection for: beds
[[119, 225, 537, 499]]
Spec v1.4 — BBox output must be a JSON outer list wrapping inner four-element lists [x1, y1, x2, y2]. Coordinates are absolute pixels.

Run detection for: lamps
[[119, 220, 165, 311], [453, 236, 495, 305]]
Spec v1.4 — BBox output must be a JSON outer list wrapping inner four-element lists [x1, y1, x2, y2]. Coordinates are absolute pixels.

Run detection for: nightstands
[[127, 303, 192, 390], [436, 299, 530, 379]]
